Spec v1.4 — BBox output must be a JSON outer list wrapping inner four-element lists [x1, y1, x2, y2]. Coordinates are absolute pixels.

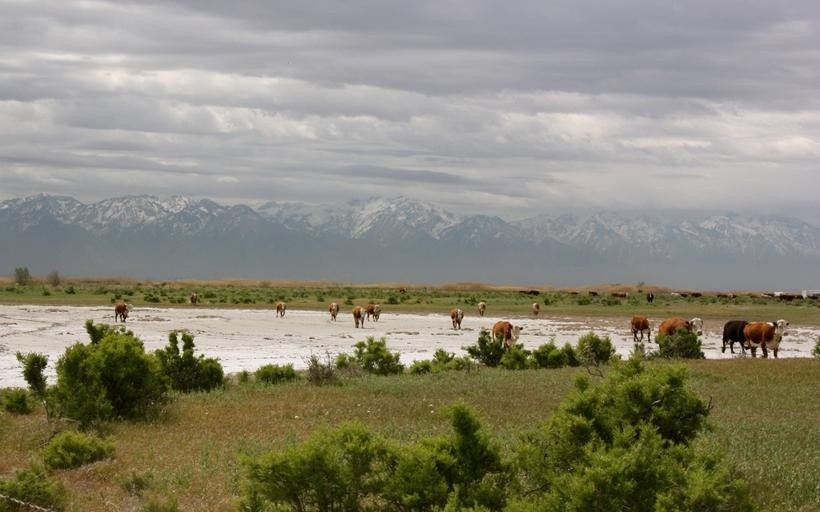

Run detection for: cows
[[722, 319, 791, 358], [275, 301, 288, 318], [531, 302, 540, 314], [352, 304, 367, 328], [366, 303, 382, 322], [114, 302, 133, 321], [477, 298, 487, 317], [491, 320, 524, 348], [450, 308, 465, 330], [630, 314, 656, 344], [657, 317, 704, 351], [327, 302, 340, 321]]
